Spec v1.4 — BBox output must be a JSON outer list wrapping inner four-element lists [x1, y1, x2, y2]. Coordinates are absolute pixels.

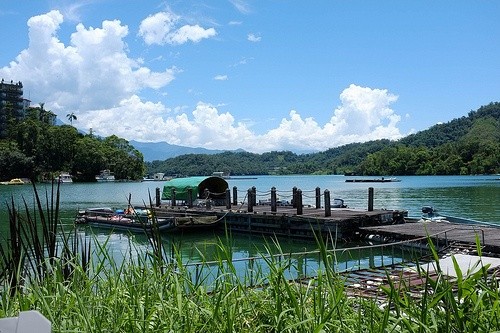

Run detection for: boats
[[55, 174, 74, 184], [148, 175, 347, 209], [143, 172, 175, 181], [75, 207, 220, 234], [345, 176, 401, 183], [94, 169, 134, 182], [421, 206, 500, 228], [212, 172, 258, 180], [0, 177, 24, 184]]
